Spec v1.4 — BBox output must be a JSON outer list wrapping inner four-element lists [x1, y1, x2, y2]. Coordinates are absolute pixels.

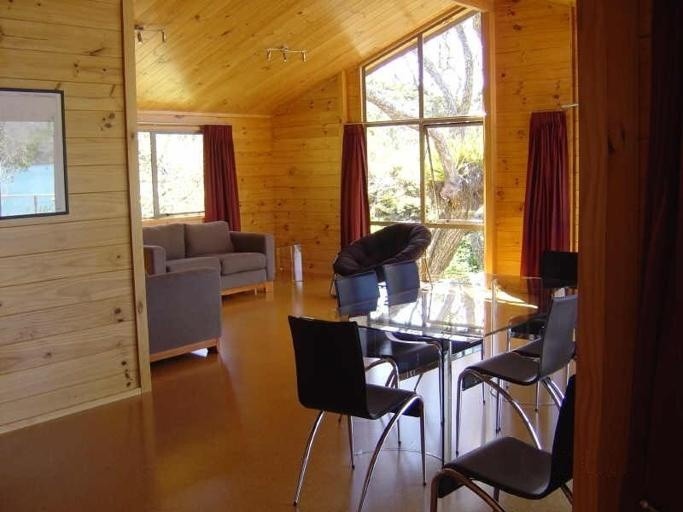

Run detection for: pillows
[[143, 221, 234, 260]]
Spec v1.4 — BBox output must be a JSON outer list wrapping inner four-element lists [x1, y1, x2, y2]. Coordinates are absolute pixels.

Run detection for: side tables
[[276, 240, 302, 281]]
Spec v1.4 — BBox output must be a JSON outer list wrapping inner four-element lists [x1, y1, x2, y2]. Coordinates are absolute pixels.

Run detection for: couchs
[[143, 221, 276, 365]]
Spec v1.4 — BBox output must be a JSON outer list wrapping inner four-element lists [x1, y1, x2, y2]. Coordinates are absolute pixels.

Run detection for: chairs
[[335, 249, 578, 470], [429, 374, 579, 511], [329, 223, 434, 298], [288, 316, 427, 511]]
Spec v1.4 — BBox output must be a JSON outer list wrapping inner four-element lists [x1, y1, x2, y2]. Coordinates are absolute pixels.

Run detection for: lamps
[[265, 48, 309, 64], [135, 27, 168, 45]]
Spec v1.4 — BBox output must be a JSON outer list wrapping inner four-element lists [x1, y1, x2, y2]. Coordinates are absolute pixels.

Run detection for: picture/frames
[[0, 88, 69, 220]]
